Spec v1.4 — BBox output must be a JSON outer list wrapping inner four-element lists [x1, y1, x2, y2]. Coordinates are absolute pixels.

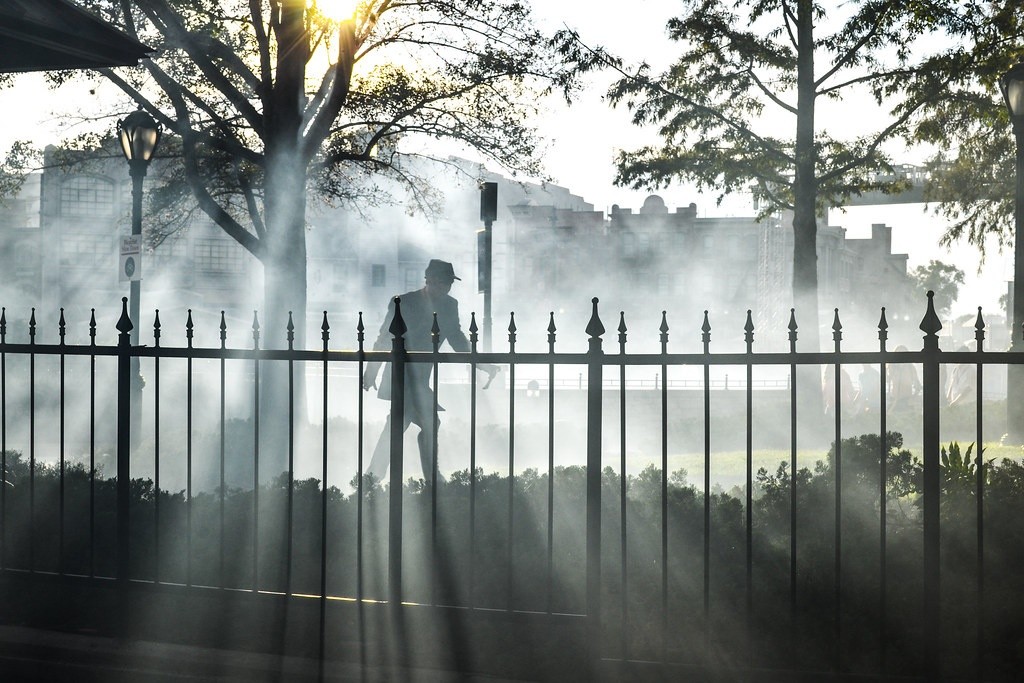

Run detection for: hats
[[427, 260, 461, 281]]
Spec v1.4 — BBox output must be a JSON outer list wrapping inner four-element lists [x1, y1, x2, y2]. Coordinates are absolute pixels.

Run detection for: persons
[[361, 259, 501, 490], [822, 345, 980, 420]]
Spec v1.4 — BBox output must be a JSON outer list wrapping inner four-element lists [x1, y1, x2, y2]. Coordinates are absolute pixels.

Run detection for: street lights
[[117, 107, 163, 477]]
